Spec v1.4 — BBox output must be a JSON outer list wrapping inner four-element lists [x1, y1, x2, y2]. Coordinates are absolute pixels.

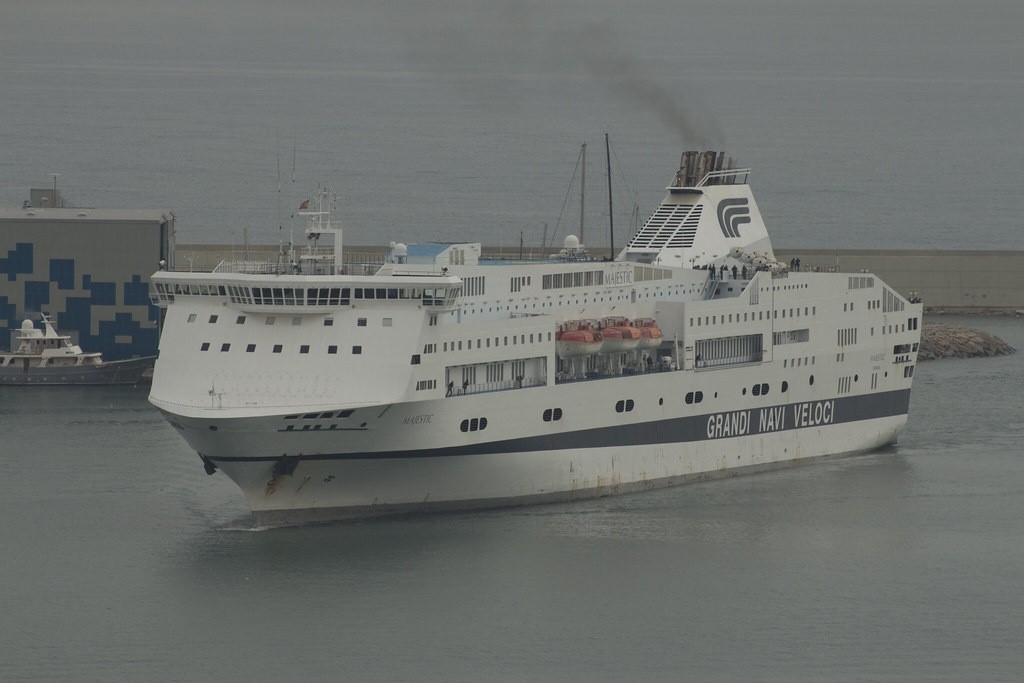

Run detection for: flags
[[299, 199, 309, 210]]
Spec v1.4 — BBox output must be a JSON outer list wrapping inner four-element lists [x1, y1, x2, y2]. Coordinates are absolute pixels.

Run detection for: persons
[[789, 258, 794, 271], [732, 265, 737, 279], [755, 264, 769, 272], [516, 374, 523, 388], [708, 264, 716, 280], [720, 265, 728, 280], [693, 265, 700, 269], [702, 264, 707, 270], [463, 379, 469, 394], [602, 255, 608, 261], [445, 381, 454, 397], [741, 265, 748, 279], [647, 355, 652, 373], [695, 354, 701, 368], [795, 258, 801, 272], [643, 355, 646, 365]]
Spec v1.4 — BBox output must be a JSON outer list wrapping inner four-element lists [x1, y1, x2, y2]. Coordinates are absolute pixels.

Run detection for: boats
[[0, 313, 157, 387], [631, 317, 664, 348], [598, 316, 641, 353], [556, 319, 605, 359]]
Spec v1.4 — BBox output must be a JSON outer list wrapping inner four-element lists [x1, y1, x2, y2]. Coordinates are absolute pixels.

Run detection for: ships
[[140, 149, 924, 534]]
